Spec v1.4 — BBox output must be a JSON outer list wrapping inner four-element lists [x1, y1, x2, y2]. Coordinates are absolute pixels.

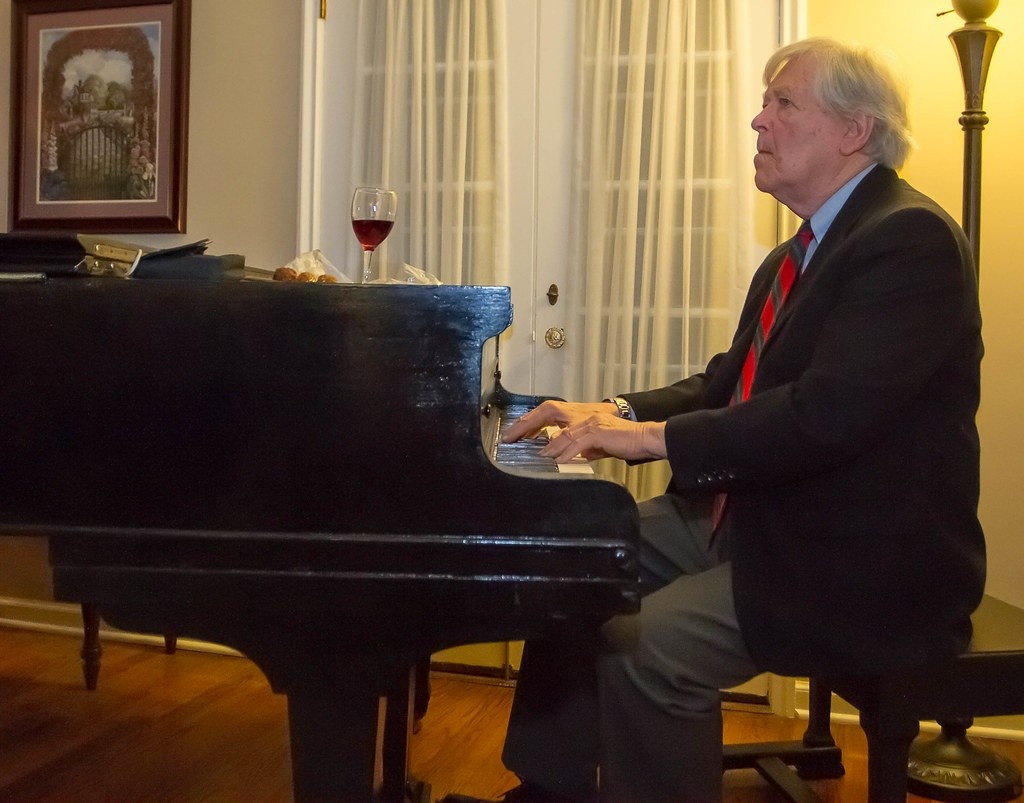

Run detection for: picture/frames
[[6, 1, 192, 236]]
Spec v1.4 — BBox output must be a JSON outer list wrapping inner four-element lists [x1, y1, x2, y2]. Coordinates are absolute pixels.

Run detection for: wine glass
[[351, 186, 398, 285]]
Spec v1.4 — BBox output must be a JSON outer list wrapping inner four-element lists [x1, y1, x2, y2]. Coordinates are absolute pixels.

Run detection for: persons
[[443, 32, 987, 803]]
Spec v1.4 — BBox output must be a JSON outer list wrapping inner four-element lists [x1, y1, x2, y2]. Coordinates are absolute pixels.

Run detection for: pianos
[[0, 231, 643, 803]]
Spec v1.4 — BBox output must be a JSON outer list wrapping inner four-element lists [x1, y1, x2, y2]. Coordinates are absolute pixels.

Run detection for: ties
[[708, 220, 815, 550]]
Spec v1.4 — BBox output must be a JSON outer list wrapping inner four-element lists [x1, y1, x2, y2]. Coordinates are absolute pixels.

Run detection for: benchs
[[723, 594, 1024, 803]]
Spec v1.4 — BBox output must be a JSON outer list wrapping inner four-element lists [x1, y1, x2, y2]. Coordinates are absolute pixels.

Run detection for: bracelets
[[601, 397, 632, 421]]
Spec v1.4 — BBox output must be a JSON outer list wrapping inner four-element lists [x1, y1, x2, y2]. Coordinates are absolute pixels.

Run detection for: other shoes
[[436, 783, 550, 803]]
